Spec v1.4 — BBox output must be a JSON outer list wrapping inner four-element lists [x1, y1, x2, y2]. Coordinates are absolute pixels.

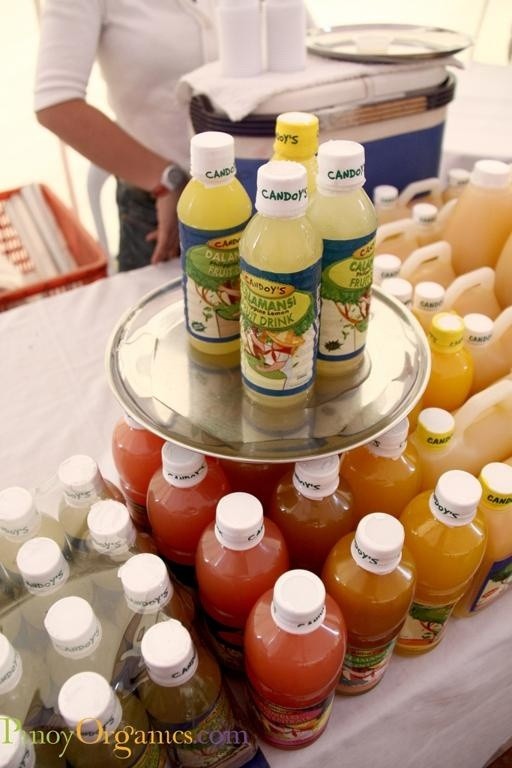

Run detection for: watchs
[[150, 163, 186, 202]]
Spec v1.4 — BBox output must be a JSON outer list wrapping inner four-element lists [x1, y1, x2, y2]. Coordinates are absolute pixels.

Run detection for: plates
[[306, 24, 469, 60], [110, 273, 431, 463]]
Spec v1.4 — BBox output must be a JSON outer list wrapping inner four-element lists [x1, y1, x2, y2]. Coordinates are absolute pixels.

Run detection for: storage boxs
[[187, 70, 457, 218]]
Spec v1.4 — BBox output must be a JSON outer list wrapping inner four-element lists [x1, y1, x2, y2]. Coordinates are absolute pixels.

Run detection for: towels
[[183, 41, 467, 130]]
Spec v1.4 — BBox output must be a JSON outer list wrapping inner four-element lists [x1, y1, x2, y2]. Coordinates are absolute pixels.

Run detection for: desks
[[2, 254, 512, 768]]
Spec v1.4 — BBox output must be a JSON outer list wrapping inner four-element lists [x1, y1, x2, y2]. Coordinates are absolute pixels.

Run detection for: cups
[[265, 0, 308, 73], [219, 2, 263, 75]]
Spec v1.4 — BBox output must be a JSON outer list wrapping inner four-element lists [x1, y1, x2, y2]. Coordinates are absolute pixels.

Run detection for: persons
[[30, 0, 326, 274]]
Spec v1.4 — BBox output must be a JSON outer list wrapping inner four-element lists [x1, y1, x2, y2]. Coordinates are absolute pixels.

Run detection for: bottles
[[375, 159, 512, 409], [0, 409, 511, 766], [312, 142, 376, 373], [266, 110, 317, 187], [177, 132, 254, 374], [239, 158, 313, 406]]
